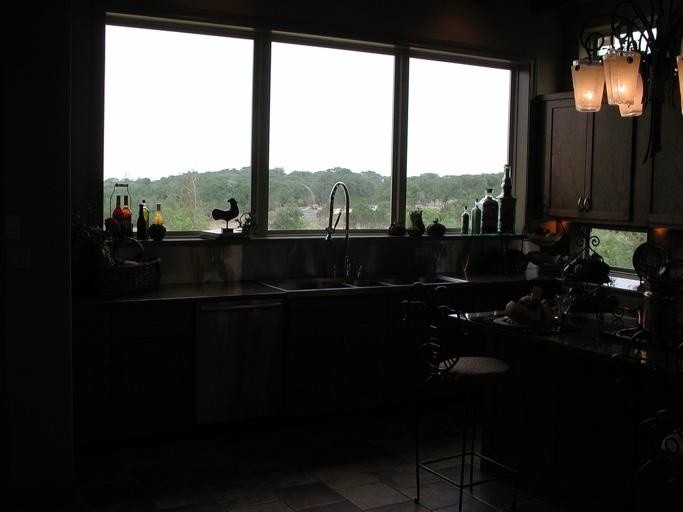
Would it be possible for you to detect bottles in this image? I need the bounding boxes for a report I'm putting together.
[112,194,132,227]
[137,197,164,240]
[460,164,516,236]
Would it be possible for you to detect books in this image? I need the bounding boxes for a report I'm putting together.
[199,229,246,243]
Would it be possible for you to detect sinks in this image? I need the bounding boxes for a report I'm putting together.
[339,277,394,289]
[257,278,356,293]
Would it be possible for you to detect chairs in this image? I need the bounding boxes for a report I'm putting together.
[398,280,533,510]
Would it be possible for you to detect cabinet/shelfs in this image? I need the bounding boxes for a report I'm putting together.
[473,290,511,430]
[285,292,386,423]
[635,80,682,230]
[73,296,197,450]
[616,323,683,512]
[386,290,472,428]
[196,298,286,428]
[606,294,645,318]
[537,92,642,228]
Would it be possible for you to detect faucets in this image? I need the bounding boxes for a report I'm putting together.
[325,181,352,280]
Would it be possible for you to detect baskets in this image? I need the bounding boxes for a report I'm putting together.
[89,238,160,293]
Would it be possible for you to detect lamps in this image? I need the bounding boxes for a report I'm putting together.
[564,1,681,117]
